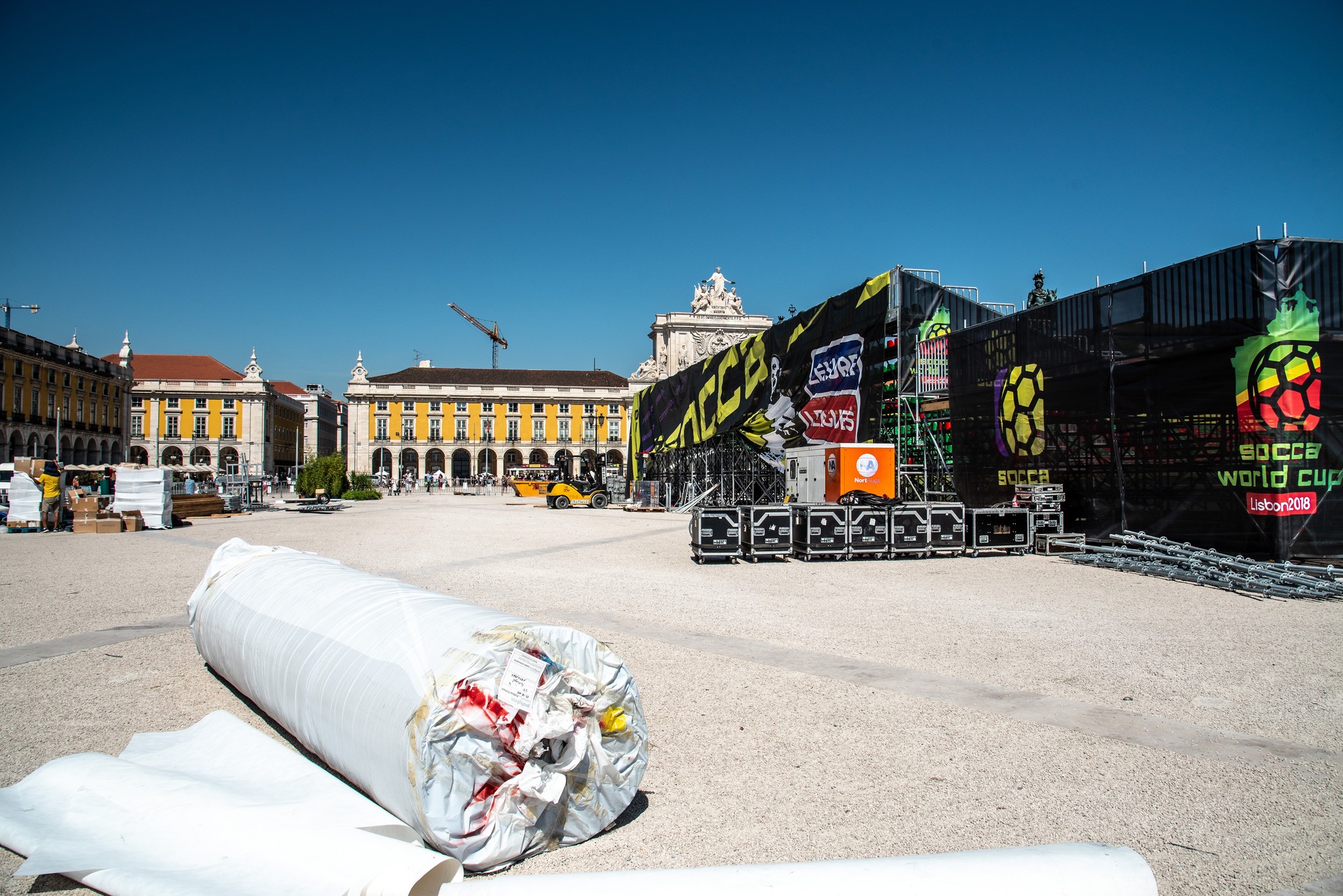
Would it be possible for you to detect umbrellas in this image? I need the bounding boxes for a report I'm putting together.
[64,463,227,482]
[479,472,493,475]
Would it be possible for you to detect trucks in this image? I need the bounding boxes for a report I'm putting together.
[784,442,895,503]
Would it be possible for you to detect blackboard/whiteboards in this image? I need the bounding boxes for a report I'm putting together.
[416,480,420,487]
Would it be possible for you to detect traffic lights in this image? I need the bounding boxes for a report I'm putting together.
[414,468,417,473]
[473,466,476,471]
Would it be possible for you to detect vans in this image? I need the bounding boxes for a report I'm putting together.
[0,462,14,503]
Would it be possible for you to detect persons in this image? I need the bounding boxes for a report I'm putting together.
[29,461,78,533]
[185,473,279,497]
[382,469,626,496]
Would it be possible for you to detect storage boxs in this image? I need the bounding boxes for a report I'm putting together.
[691,483,1087,565]
[6,456,142,534]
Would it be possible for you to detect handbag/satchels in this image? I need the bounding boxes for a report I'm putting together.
[267,486,272,493]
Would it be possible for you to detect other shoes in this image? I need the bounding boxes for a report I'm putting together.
[262,493,264,496]
[471,485,498,487]
[275,490,278,493]
[387,493,412,496]
[426,491,431,492]
[267,495,270,496]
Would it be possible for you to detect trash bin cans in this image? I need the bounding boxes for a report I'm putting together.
[425,479,427,487]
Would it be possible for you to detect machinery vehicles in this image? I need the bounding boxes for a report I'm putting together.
[546,453,631,510]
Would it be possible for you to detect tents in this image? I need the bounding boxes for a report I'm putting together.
[433,470,445,476]
[375,470,390,479]
[585,471,595,481]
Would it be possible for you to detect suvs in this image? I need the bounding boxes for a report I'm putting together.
[358,475,388,487]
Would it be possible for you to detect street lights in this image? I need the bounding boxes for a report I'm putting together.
[561,437,572,458]
[588,408,605,454]
[395,432,408,487]
[192,430,197,475]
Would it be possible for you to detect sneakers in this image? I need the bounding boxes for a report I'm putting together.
[53,528,59,532]
[40,528,49,533]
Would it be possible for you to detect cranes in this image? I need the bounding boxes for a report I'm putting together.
[447,302,509,369]
